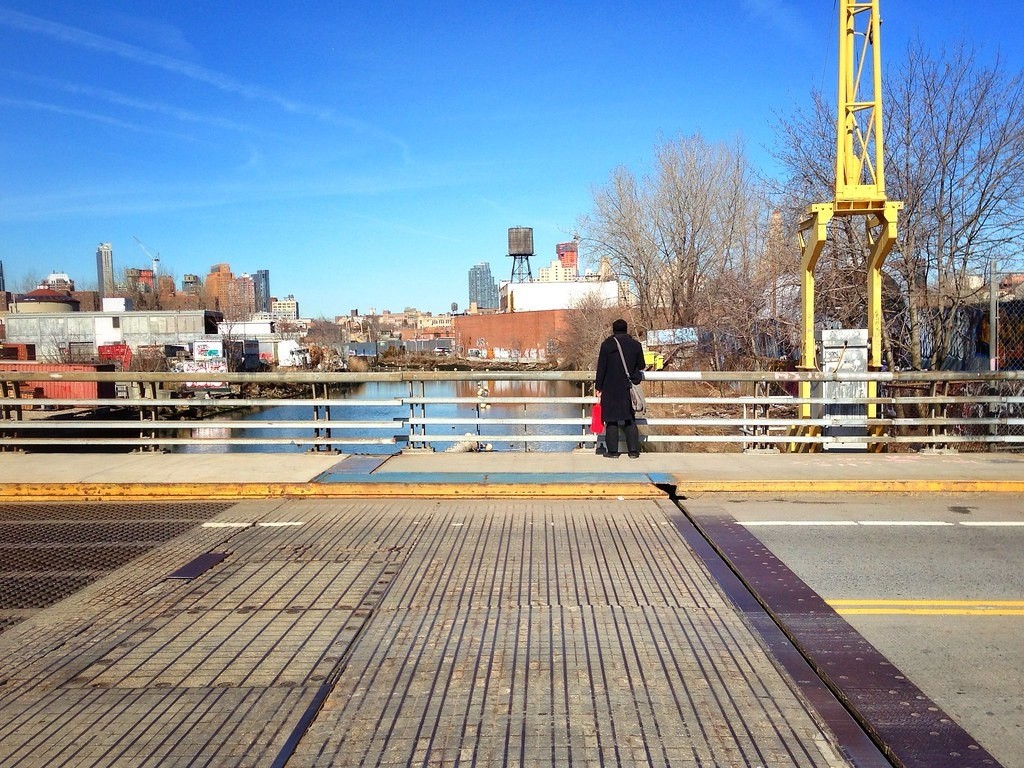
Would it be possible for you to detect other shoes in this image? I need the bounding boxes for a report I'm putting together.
[627,451,640,458]
[603,451,619,458]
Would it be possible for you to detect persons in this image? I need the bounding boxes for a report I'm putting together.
[595,319,646,458]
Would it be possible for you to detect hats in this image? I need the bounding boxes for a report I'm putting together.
[612,319,628,332]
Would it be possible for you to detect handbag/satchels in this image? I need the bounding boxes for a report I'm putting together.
[589,396,605,434]
[630,382,646,415]
